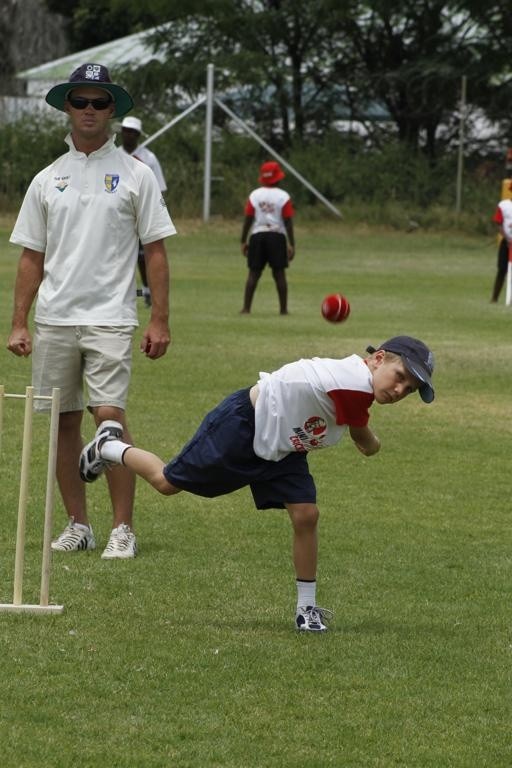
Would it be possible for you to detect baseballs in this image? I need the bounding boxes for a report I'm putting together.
[322,295,351,322]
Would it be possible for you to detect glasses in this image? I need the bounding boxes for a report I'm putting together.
[67,96,112,111]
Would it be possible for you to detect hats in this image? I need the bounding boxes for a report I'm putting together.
[46,63,135,119]
[112,116,150,139]
[377,335,434,404]
[258,161,286,185]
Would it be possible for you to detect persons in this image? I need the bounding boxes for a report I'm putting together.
[78,336,435,633]
[7,63,178,561]
[239,161,295,315]
[489,184,511,302]
[111,115,169,308]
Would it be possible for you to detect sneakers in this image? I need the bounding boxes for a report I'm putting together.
[100,523,141,559]
[78,420,124,482]
[294,604,334,631]
[51,517,97,553]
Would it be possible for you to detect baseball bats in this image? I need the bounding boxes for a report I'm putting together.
[506,243,511,305]
